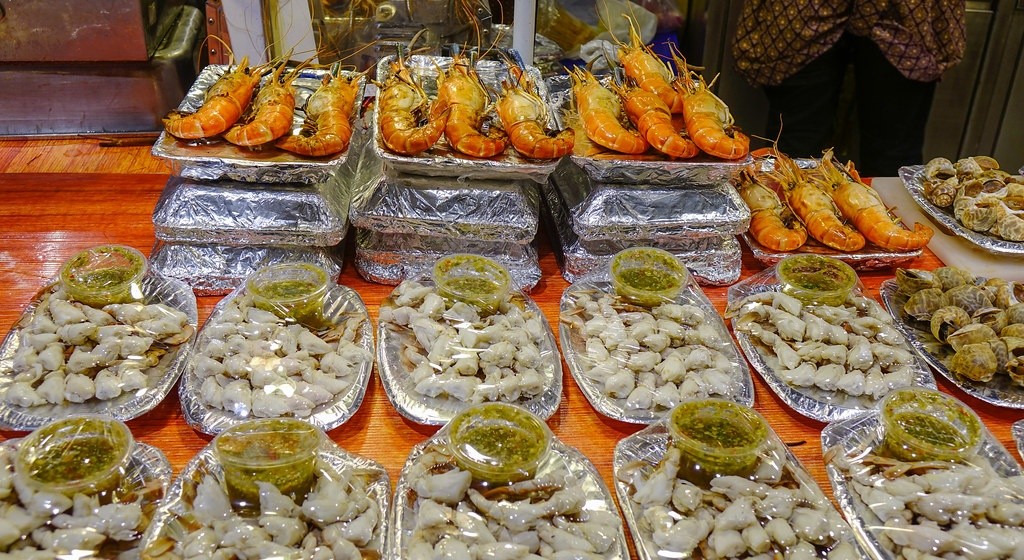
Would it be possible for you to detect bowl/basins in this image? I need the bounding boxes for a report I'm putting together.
[610,246,689,306]
[213,417,322,511]
[58,245,150,307]
[245,261,331,324]
[670,398,770,486]
[777,254,857,306]
[874,386,985,465]
[14,414,135,499]
[447,402,554,486]
[432,253,510,318]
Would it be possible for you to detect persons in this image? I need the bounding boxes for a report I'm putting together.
[731,0,967,178]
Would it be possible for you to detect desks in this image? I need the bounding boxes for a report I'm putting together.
[0,170,1024,560]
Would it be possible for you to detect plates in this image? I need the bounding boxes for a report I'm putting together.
[730,284,1024,559]
[178,285,374,430]
[137,437,390,559]
[380,288,561,425]
[152,63,1023,296]
[390,427,631,559]
[883,276,1024,462]
[561,278,867,559]
[0,434,172,560]
[0,276,200,431]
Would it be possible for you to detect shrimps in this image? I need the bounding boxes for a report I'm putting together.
[731,112,935,252]
[160,0,751,159]
